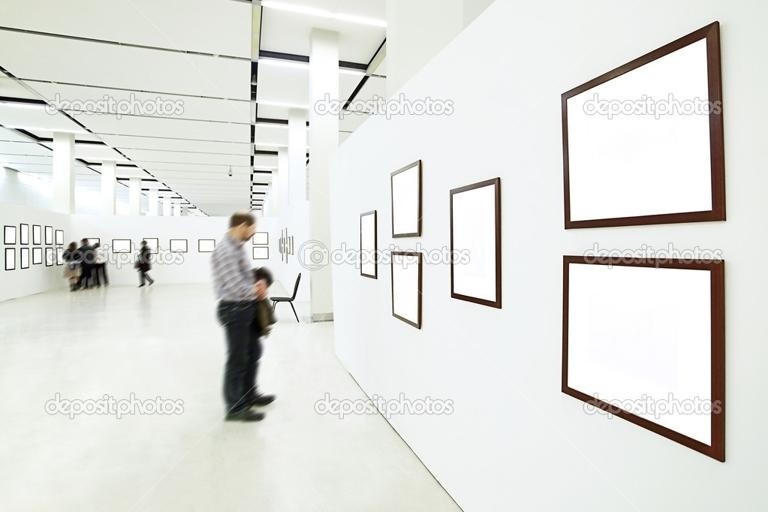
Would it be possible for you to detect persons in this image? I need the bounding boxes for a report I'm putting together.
[59,237,112,293]
[208,211,277,423]
[133,239,156,289]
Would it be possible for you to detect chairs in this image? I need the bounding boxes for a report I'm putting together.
[264,271,300,325]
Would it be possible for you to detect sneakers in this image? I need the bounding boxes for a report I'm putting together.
[226,409,264,421]
[245,395,274,407]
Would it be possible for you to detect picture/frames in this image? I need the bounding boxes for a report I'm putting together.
[449,177,501,308]
[86,238,217,255]
[391,251,419,329]
[561,21,728,230]
[391,160,422,237]
[4,222,64,270]
[250,228,294,265]
[561,256,725,461]
[359,210,378,279]
